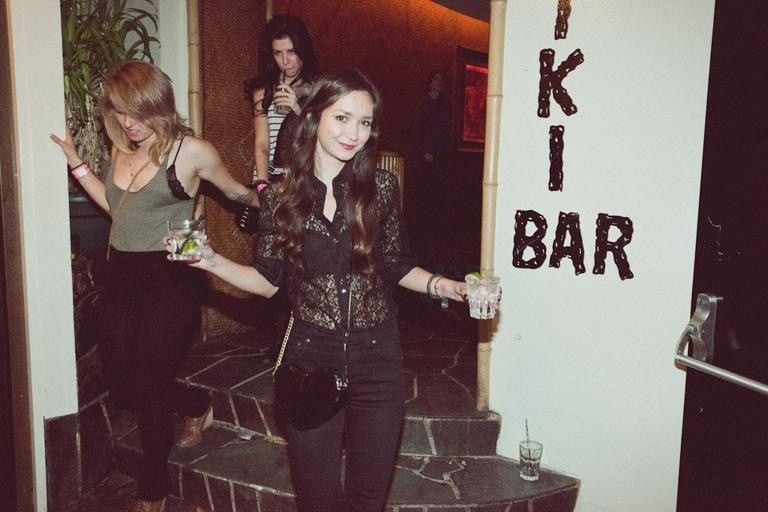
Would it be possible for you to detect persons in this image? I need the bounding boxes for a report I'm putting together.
[49,58,259,512]
[244,14,316,193]
[162,67,502,511]
[411,69,452,172]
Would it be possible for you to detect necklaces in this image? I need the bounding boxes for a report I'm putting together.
[128,154,149,178]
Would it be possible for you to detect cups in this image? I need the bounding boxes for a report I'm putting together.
[165,217,206,264]
[464,272,501,321]
[517,441,543,482]
[272,84,293,114]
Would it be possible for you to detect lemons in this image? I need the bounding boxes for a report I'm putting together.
[183,241,199,253]
[464,271,482,284]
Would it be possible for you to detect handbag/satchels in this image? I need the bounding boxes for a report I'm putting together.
[273,363,350,432]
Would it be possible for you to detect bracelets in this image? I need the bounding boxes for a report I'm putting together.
[255,179,268,193]
[434,276,449,310]
[71,161,90,179]
[426,273,441,297]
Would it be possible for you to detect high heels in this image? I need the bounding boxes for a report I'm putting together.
[173,398,215,448]
[130,497,167,512]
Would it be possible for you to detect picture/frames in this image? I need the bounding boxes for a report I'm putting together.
[454,44,489,155]
